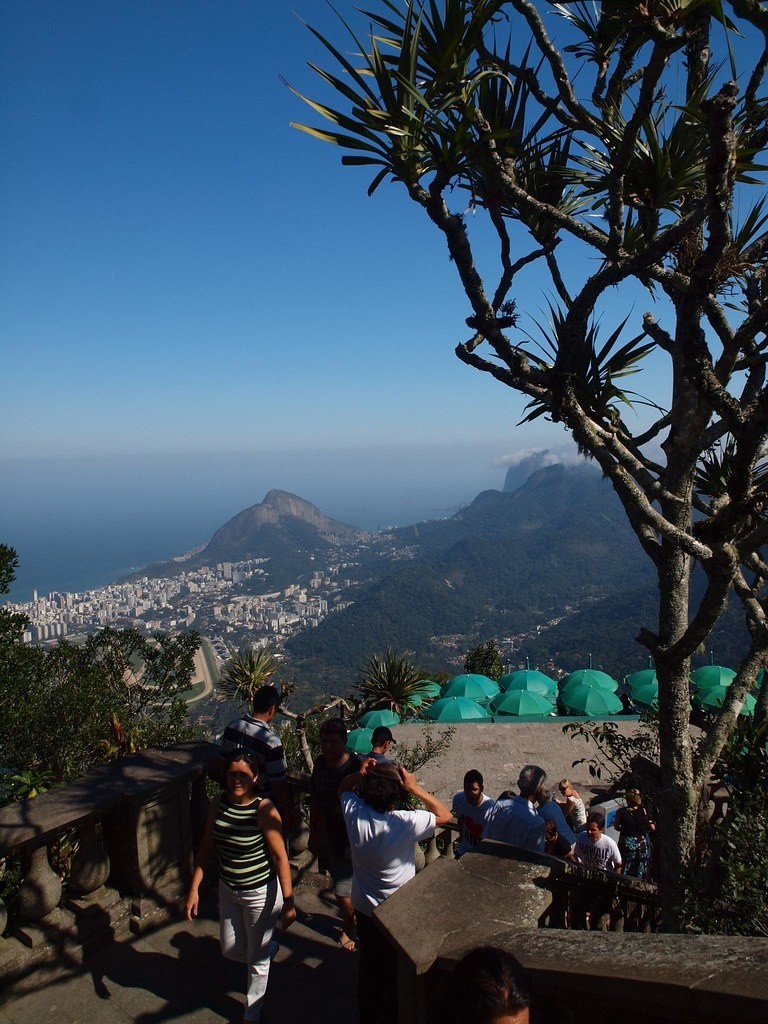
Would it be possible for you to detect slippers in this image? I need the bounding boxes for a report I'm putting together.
[338,922,356,952]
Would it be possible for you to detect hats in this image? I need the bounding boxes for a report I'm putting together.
[373,726,396,744]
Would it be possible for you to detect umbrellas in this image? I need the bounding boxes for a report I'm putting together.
[557,669,623,715]
[347,710,400,754]
[402,670,559,720]
[624,669,659,707]
[689,666,737,686]
[692,685,757,717]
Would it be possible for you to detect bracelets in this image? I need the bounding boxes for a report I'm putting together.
[284,896,294,901]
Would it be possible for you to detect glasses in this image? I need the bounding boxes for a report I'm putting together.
[229,749,255,762]
[560,786,568,790]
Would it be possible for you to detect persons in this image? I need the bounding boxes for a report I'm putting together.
[308,718,359,950]
[615,789,656,878]
[573,813,622,874]
[358,726,399,765]
[187,751,297,1024]
[433,947,534,1024]
[483,765,547,854]
[221,685,289,828]
[451,770,495,858]
[337,756,453,1024]
[497,790,516,800]
[536,780,587,860]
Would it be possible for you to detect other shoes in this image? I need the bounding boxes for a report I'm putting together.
[268,941,279,959]
